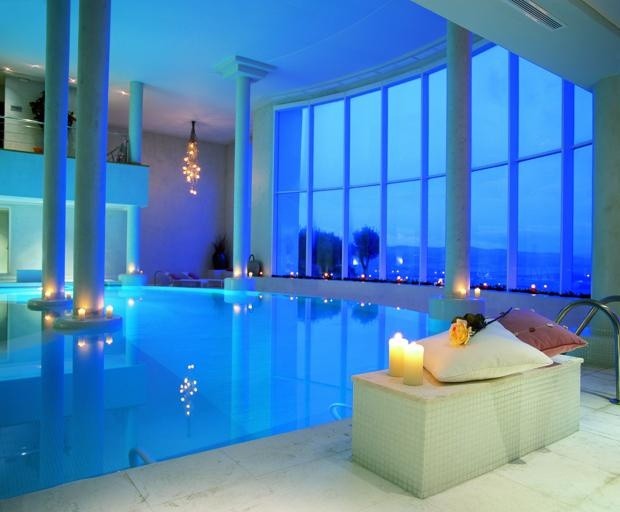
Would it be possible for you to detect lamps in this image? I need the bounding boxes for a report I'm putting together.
[177,363,199,433]
[182,121,202,195]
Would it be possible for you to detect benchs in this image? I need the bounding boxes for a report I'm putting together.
[351,356,585,498]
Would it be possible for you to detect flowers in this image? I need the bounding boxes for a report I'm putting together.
[448,308,513,348]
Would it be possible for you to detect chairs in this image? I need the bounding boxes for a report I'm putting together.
[154,270,223,288]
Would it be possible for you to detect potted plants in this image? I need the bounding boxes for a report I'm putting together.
[211,233,231,270]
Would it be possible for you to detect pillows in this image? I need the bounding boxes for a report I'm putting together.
[413,318,553,383]
[496,309,587,356]
[172,272,199,280]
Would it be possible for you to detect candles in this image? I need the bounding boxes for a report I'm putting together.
[474,288,480,297]
[67,291,71,298]
[389,338,408,377]
[106,333,113,344]
[403,342,424,387]
[106,305,112,317]
[78,335,86,348]
[44,312,50,319]
[78,308,86,320]
